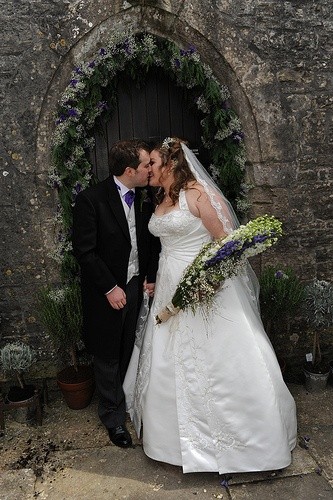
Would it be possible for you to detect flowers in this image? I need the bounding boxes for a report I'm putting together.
[303,279,333,330]
[156,213,283,324]
[0,342,44,377]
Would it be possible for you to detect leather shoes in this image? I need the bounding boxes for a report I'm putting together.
[109,424,132,448]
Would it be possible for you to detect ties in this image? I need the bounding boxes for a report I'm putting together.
[115,184,136,208]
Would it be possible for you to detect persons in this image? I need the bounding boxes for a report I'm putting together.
[122,136,297,474]
[72,138,162,448]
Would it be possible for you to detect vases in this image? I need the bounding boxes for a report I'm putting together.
[305,365,329,392]
[5,385,41,424]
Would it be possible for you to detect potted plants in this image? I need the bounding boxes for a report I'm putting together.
[258,265,305,383]
[37,252,95,411]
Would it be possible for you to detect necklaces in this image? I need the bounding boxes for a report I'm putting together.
[164,193,170,202]
